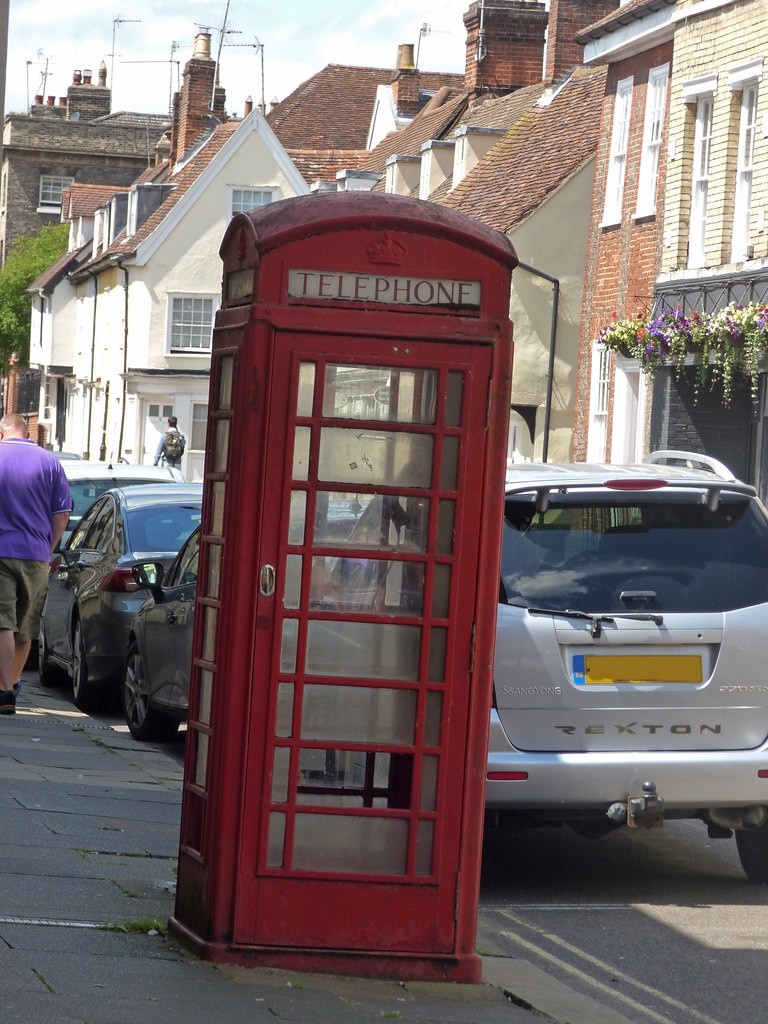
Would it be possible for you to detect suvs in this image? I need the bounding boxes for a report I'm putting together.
[309,448,767,883]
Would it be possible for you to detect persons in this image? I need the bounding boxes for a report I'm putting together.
[0,413,73,716]
[154,416,185,471]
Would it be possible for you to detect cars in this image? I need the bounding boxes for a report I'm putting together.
[120,505,367,743]
[36,482,202,713]
[41,462,186,552]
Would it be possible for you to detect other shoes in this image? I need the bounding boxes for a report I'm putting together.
[0,690,16,715]
[12,680,21,693]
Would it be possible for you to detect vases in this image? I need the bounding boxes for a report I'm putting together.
[708,336,724,349]
[666,344,686,356]
[726,332,745,349]
[618,341,636,358]
[687,339,704,354]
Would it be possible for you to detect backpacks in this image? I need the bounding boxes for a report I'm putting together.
[163,432,186,459]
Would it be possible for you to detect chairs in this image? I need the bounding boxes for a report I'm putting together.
[598,524,650,563]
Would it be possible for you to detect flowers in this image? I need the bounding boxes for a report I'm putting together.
[598,301,768,414]
